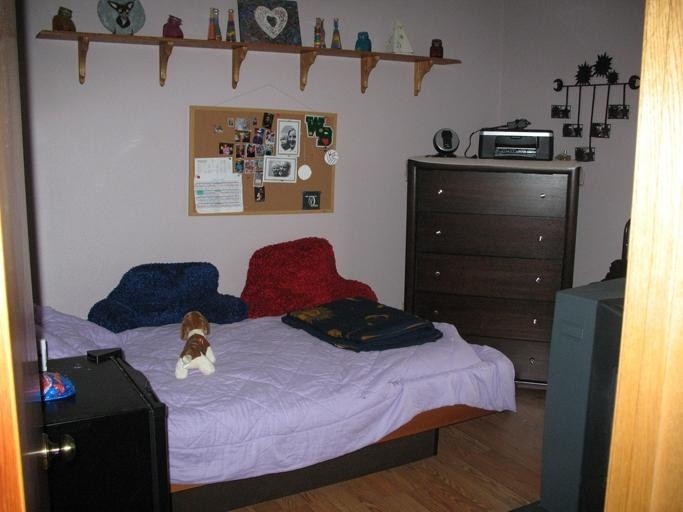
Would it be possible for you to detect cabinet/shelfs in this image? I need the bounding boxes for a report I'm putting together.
[405,155,581,390]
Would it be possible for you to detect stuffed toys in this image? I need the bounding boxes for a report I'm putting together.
[174,311,215,379]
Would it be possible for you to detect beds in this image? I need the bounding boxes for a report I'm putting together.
[35,298,518,512]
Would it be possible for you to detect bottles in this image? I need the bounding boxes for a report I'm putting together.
[331,17,342,49]
[51,4,76,30]
[355,32,371,52]
[225,8,236,43]
[320,20,327,49]
[314,17,322,49]
[162,14,184,40]
[212,10,222,41]
[207,8,214,40]
[429,39,446,58]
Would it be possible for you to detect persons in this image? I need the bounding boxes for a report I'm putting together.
[271,162,280,176]
[280,161,291,177]
[279,134,290,150]
[287,129,297,152]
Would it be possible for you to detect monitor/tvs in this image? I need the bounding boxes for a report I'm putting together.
[539,277,625,512]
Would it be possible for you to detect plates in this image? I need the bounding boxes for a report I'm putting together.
[95,1,145,37]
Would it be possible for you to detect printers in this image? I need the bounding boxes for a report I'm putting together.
[479,129,554,160]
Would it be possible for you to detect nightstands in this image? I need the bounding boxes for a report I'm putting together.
[40,349,171,511]
